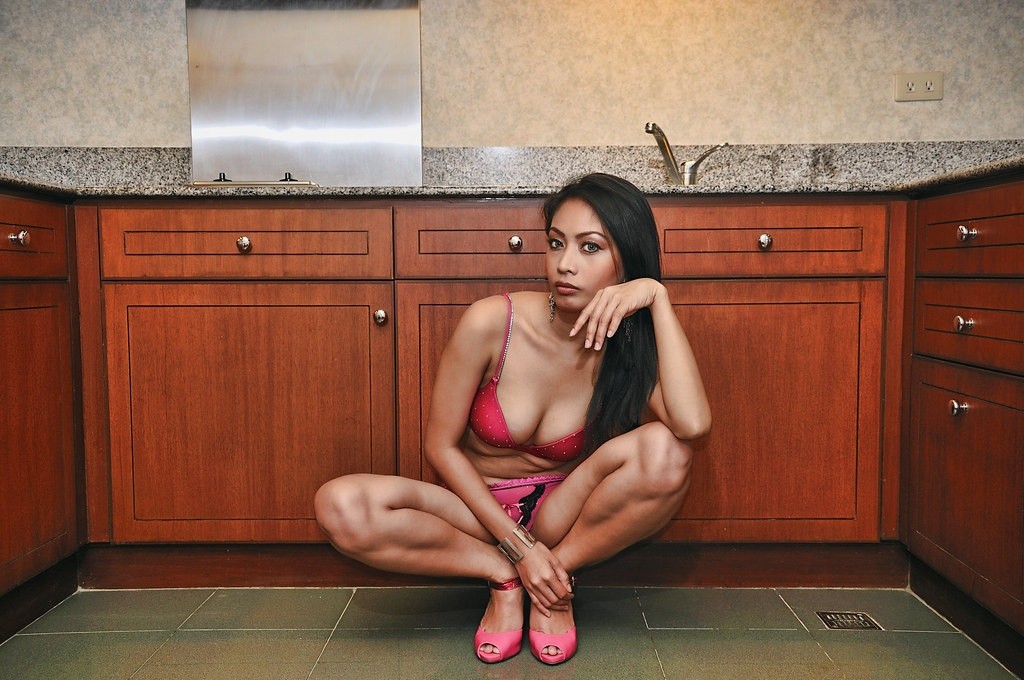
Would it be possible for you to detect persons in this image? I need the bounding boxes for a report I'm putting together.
[312,173,712,666]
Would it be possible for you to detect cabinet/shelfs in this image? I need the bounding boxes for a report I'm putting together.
[0,165,1024,680]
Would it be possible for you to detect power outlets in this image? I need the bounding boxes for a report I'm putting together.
[894,72,945,99]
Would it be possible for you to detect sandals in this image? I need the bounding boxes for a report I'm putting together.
[529,576,577,664]
[474,576,524,663]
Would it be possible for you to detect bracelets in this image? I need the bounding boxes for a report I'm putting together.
[497,524,537,565]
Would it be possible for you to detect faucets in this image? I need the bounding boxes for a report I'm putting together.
[646,121,729,185]
